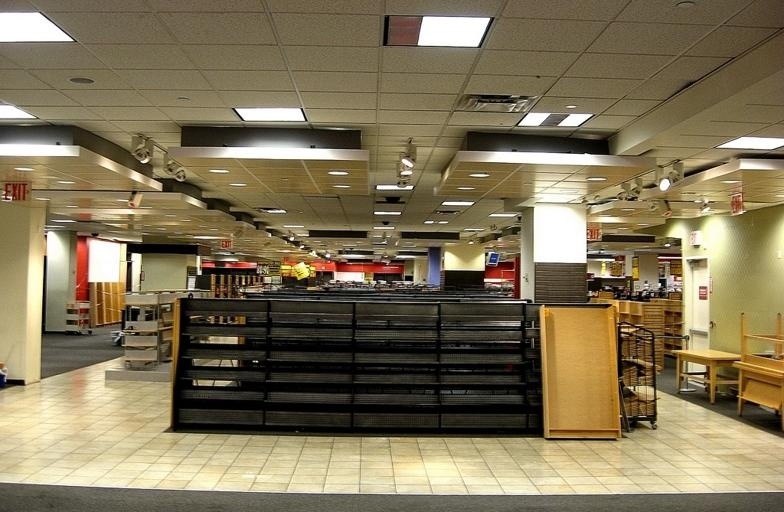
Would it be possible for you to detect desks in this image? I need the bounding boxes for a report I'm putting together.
[730,311,784,431]
[671,349,741,405]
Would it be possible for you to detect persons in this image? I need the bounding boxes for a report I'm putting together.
[374,279,397,288]
[598,280,681,302]
[334,280,340,288]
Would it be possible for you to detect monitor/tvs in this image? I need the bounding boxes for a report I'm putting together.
[486,251,500,265]
[263,275,317,288]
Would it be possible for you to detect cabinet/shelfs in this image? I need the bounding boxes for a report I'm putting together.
[89,282,542,437]
[591,297,683,386]
[65,303,92,334]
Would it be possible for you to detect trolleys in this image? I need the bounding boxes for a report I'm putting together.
[64,300,94,335]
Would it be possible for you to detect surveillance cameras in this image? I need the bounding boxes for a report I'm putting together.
[518,217,521,220]
[383,222,389,225]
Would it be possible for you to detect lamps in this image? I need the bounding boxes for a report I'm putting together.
[397,143,417,177]
